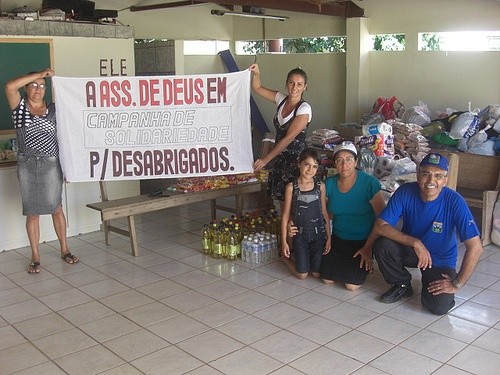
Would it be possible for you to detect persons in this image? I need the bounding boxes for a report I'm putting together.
[288,142,386,291]
[279,147,331,278]
[250,63,313,252]
[373,152,482,314]
[4,68,79,274]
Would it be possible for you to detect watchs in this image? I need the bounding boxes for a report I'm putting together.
[452,280,461,288]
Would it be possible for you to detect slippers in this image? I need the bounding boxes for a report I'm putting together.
[28,262,40,273]
[62,252,79,264]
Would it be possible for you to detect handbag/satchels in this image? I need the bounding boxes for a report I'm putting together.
[448,102,481,140]
[468,124,492,147]
[374,96,397,120]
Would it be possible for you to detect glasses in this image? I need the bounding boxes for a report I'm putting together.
[419,171,447,181]
[334,157,356,166]
[27,82,45,88]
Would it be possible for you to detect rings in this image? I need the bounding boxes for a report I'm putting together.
[369,260,371,261]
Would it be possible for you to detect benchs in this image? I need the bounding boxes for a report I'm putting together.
[86,181,262,256]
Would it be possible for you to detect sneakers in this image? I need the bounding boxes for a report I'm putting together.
[380,282,413,303]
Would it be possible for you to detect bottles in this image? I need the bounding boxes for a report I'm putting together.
[201,210,281,265]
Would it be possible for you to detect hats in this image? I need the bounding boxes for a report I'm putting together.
[420,152,449,172]
[333,141,357,156]
